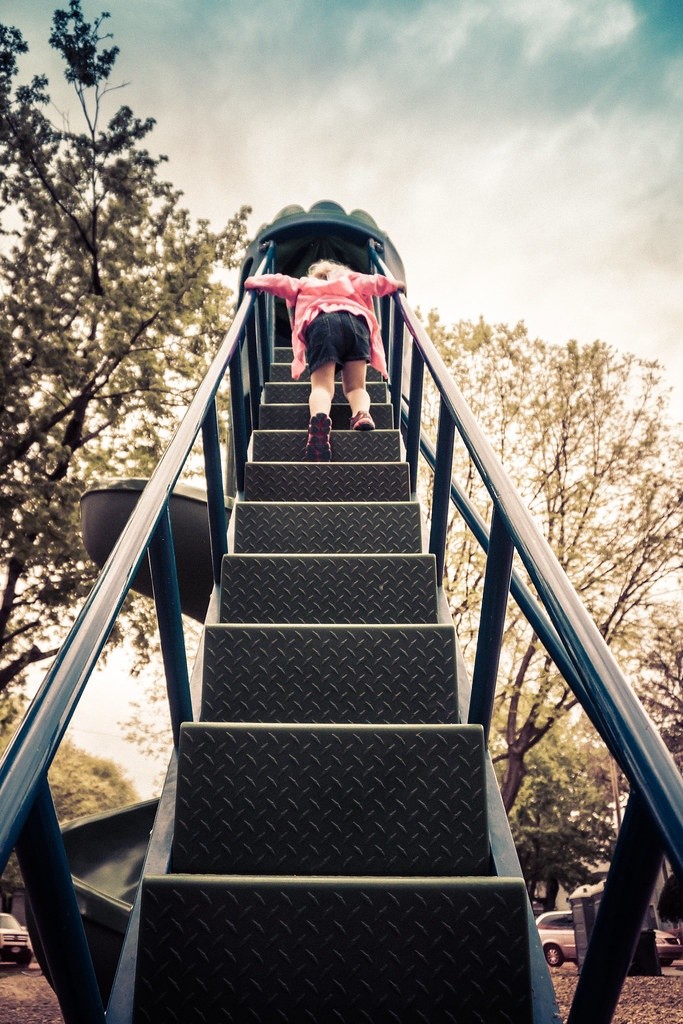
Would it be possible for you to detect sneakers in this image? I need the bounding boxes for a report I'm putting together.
[349,411,376,430]
[305,412,333,462]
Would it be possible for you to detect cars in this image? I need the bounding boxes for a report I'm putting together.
[535,910,683,967]
[0,913,33,967]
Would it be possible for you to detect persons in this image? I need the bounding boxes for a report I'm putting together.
[243,259,409,464]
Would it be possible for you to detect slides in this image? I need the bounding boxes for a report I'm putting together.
[19,480,238,979]
